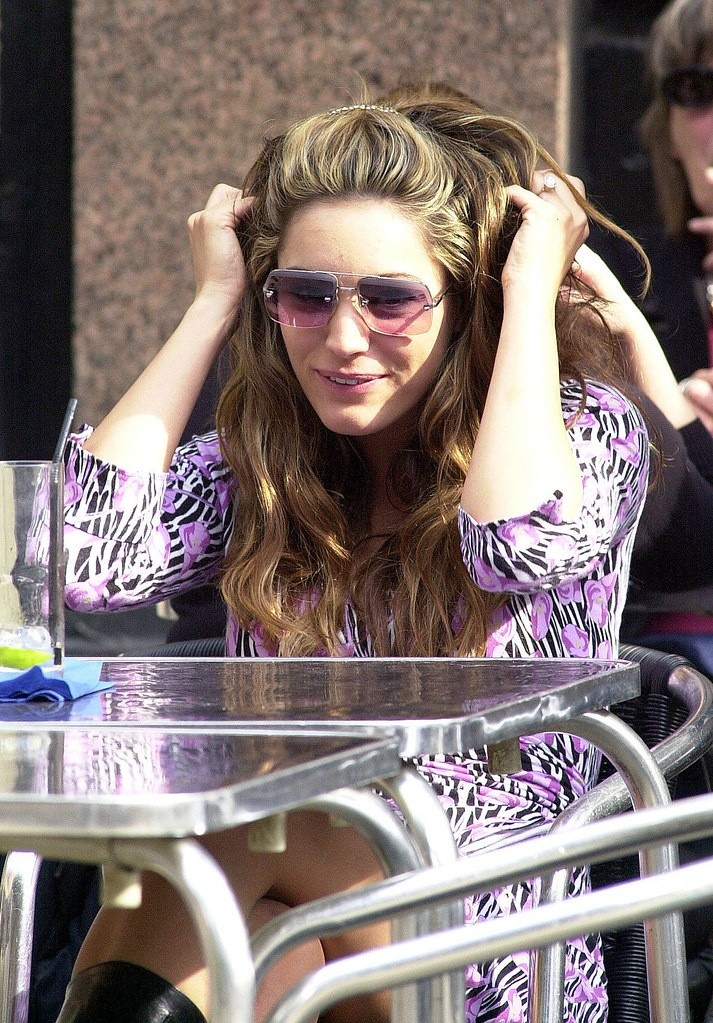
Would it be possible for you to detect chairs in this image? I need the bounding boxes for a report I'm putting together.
[28,637,712,1022]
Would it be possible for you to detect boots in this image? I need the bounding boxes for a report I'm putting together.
[56,959,208,1022]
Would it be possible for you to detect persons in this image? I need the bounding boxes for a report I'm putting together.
[24,95,653,1023]
[552,0,713,1002]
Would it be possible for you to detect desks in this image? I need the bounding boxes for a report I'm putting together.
[0,654,690,1023]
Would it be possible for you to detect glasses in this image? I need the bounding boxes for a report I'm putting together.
[263,267,455,338]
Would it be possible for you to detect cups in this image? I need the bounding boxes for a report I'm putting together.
[0,461,65,673]
